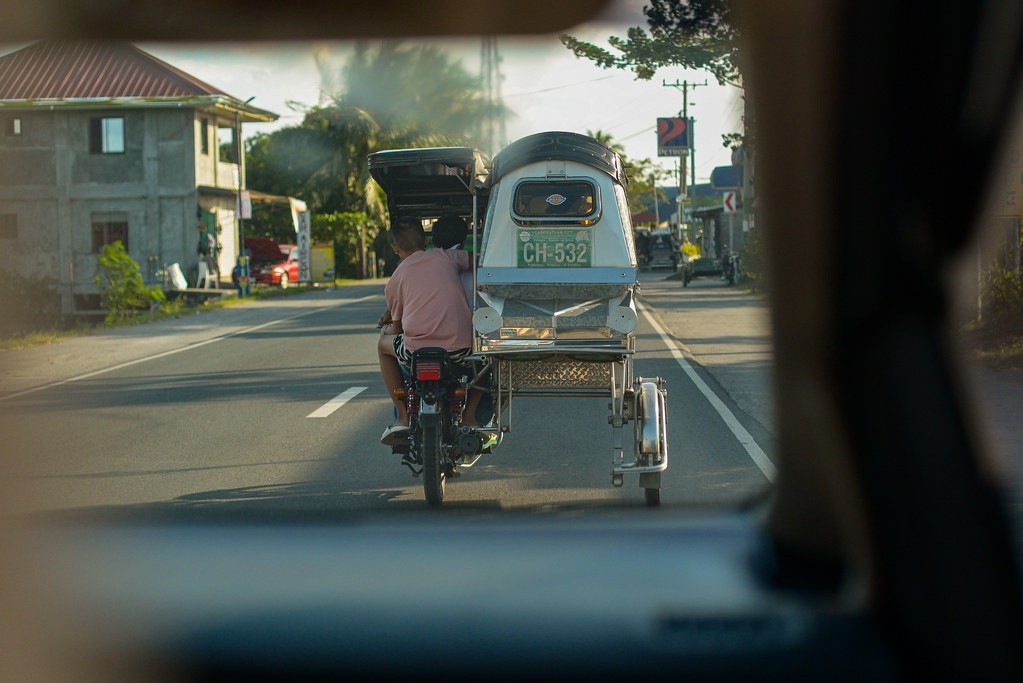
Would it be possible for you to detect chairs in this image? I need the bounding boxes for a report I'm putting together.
[195,261,219,289]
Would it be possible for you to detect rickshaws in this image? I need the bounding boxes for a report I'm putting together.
[366,130,669,506]
[633,226,739,289]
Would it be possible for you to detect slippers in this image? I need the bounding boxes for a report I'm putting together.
[379,424,410,445]
[484,433,498,448]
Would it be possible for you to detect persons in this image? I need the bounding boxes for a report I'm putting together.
[635,233,653,265]
[196,223,215,290]
[378,214,498,467]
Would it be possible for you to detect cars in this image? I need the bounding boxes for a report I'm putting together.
[244,238,299,290]
